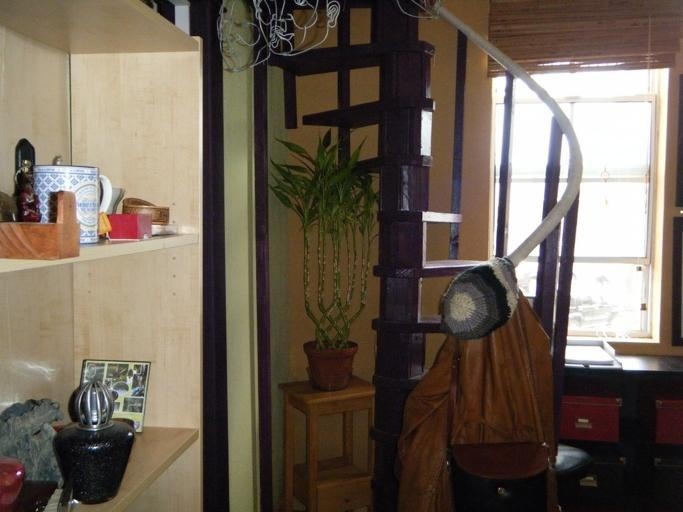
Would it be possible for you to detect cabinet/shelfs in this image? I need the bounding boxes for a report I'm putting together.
[276,375,377,511]
[0,2,206,511]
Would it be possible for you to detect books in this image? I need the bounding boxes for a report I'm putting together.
[551,343,613,366]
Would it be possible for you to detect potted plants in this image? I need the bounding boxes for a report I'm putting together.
[266,123,391,391]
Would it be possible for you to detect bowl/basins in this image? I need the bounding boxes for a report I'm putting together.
[0,457,25,512]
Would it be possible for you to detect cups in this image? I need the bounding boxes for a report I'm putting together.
[31,162,113,246]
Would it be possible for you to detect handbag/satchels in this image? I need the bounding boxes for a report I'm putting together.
[445,439,553,512]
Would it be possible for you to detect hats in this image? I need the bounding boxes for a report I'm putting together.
[438,255,520,341]
